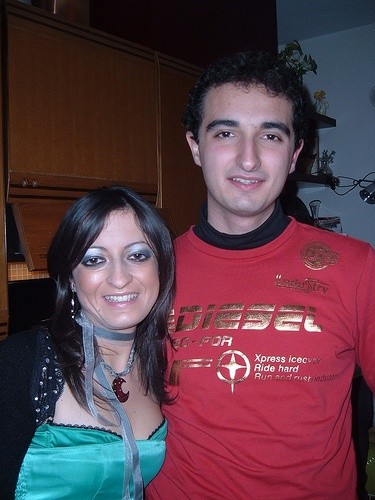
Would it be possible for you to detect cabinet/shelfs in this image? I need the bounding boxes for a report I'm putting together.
[0,0,341,343]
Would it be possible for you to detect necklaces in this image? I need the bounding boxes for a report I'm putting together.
[97,338,137,403]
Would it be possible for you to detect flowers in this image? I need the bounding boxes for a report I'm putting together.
[312,91,326,99]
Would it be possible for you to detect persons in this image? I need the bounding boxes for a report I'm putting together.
[0,185,176,499]
[143,50,374,500]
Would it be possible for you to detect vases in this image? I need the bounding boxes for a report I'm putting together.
[316,158,334,176]
[313,99,329,115]
